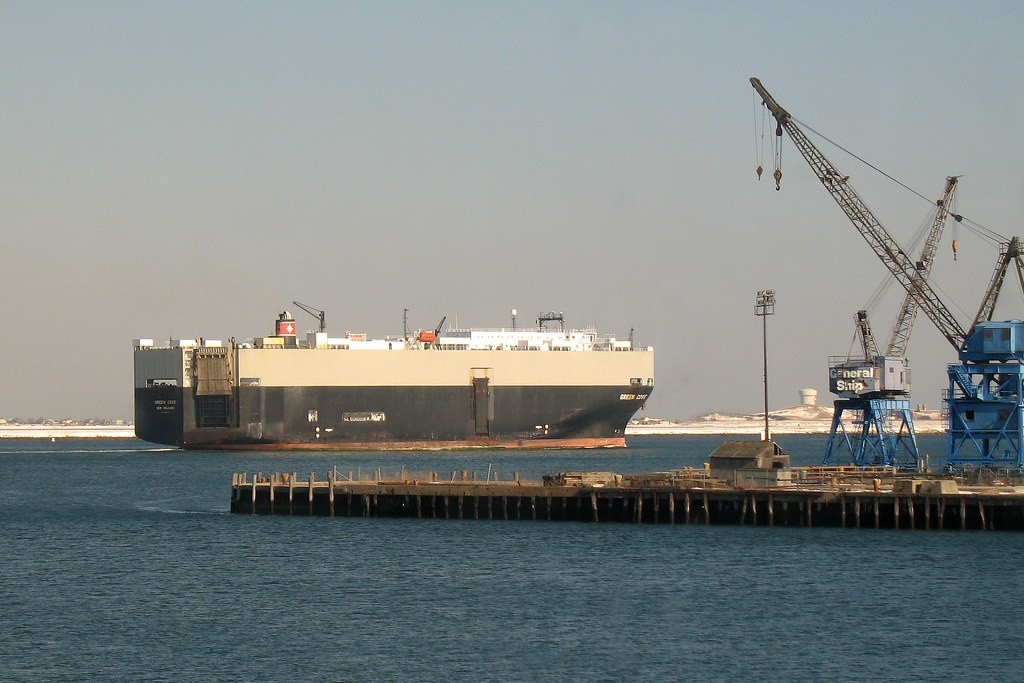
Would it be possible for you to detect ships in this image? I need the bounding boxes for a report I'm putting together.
[130,299,655,452]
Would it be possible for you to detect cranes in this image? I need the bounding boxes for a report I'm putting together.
[746,76,1024,477]
